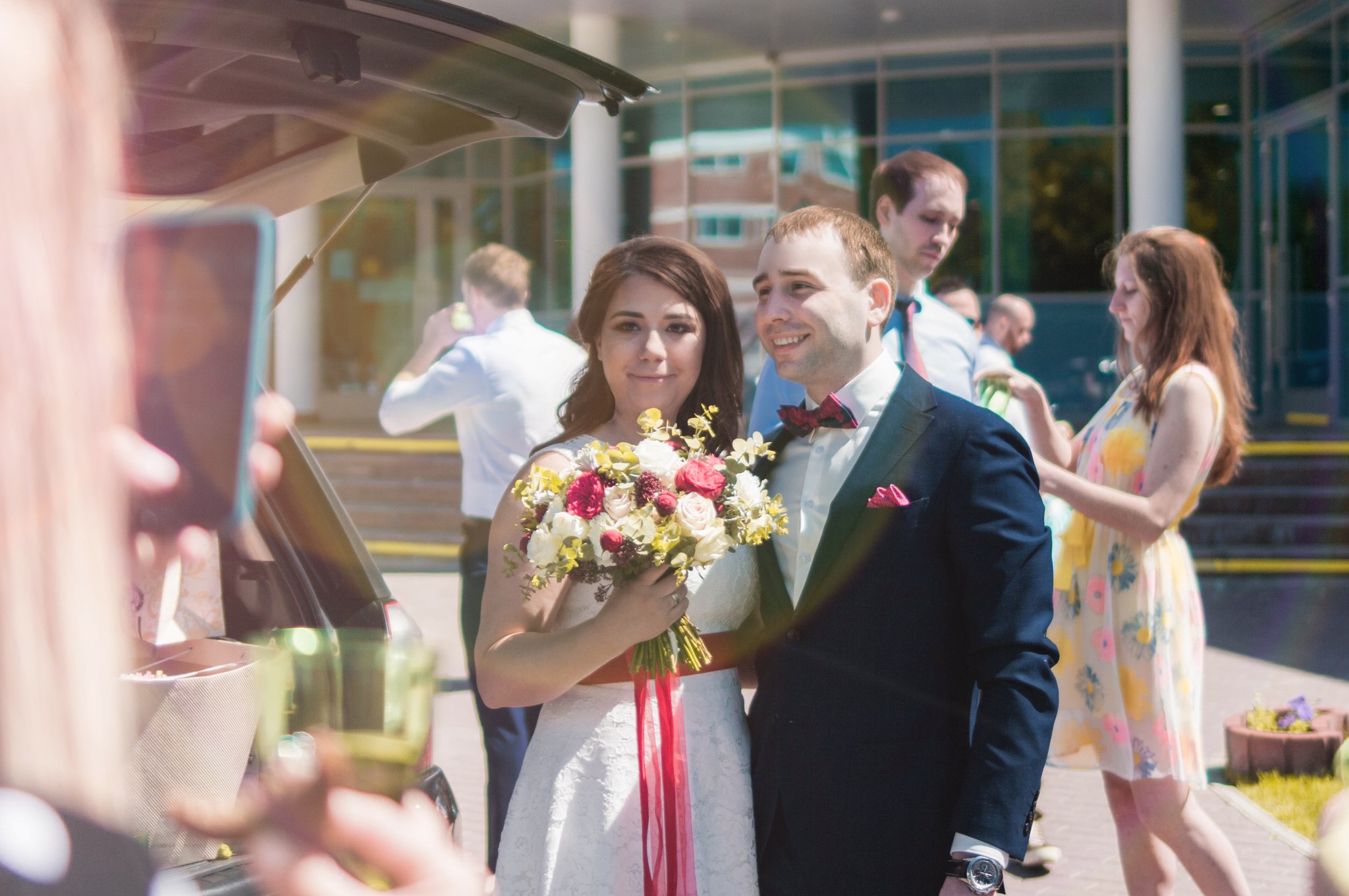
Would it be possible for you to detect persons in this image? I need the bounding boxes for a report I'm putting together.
[377,244,589,878]
[741,205,1060,896]
[0,0,459,896]
[473,232,761,896]
[736,147,1072,571]
[974,222,1258,896]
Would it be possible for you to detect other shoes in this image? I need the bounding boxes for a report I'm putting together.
[1008,810,1061,866]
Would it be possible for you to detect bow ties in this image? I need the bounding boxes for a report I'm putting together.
[776,392,858,438]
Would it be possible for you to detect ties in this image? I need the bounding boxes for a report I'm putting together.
[895,299,930,383]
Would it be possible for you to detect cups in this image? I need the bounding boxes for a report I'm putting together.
[252,627,436,891]
[452,302,474,332]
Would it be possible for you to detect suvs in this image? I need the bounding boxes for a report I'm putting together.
[107,0,658,895]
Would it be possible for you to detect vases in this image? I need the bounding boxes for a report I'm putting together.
[1224,708,1349,784]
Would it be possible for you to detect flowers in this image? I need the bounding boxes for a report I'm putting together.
[493,403,790,675]
[1245,691,1316,733]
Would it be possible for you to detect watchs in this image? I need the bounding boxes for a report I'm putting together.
[944,855,1003,895]
